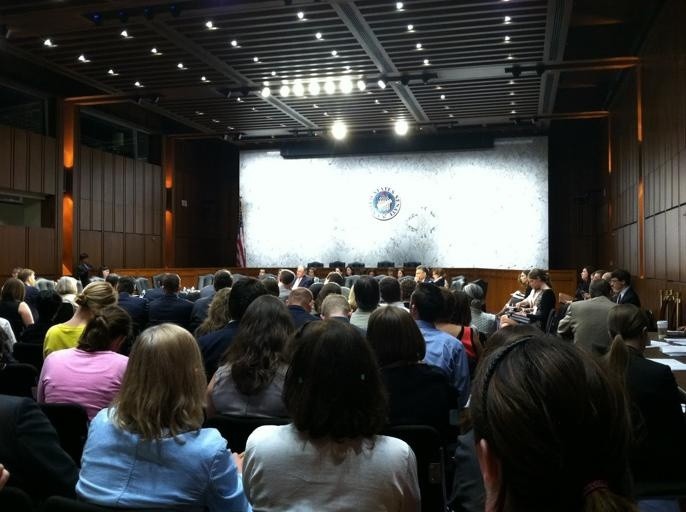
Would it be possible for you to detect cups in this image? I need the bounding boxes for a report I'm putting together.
[657,320,668,342]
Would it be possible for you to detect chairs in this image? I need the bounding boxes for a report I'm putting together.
[37,401,89,468]
[546,307,557,335]
[376,260,395,268]
[379,423,449,512]
[12,342,44,373]
[403,261,422,268]
[329,261,346,268]
[203,414,292,454]
[307,260,324,267]
[8,362,40,400]
[347,262,365,269]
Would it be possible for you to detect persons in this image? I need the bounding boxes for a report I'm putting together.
[148,273,195,323]
[345,266,354,276]
[21,290,62,343]
[415,265,434,285]
[611,269,641,306]
[335,266,343,275]
[497,270,530,314]
[557,279,626,346]
[17,268,40,303]
[258,269,268,277]
[499,273,527,314]
[283,287,322,326]
[75,324,251,512]
[242,320,422,512]
[591,273,595,281]
[315,282,342,316]
[0,327,74,512]
[463,284,497,333]
[409,283,471,405]
[42,281,124,358]
[429,268,445,287]
[0,278,34,324]
[368,269,377,276]
[350,276,383,323]
[500,268,554,329]
[37,305,132,421]
[578,267,594,299]
[196,278,275,380]
[309,268,319,284]
[205,295,298,416]
[144,274,171,299]
[472,333,686,512]
[118,277,149,322]
[594,271,605,281]
[100,265,112,279]
[0,317,17,352]
[192,287,234,338]
[293,265,313,290]
[77,253,96,279]
[365,306,461,426]
[399,278,416,302]
[398,269,405,279]
[603,304,686,498]
[300,294,372,361]
[323,273,342,287]
[194,271,235,323]
[106,275,119,291]
[601,270,612,282]
[380,277,410,312]
[278,269,294,300]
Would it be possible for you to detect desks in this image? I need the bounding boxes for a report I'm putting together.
[641,328,686,398]
